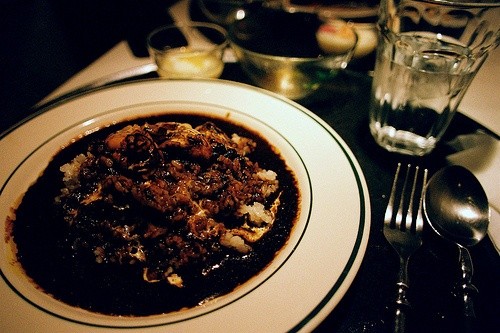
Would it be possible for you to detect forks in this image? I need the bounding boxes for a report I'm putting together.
[384,162,429,333]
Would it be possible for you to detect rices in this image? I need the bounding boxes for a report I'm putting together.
[57,121,278,289]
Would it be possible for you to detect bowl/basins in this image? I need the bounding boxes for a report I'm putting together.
[228,10,358,100]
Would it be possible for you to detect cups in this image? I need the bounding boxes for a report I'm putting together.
[146,22,229,79]
[369,0,500,157]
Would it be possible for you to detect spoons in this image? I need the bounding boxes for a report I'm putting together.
[423,164,490,333]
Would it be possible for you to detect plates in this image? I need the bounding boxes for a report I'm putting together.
[1,77,371,331]
